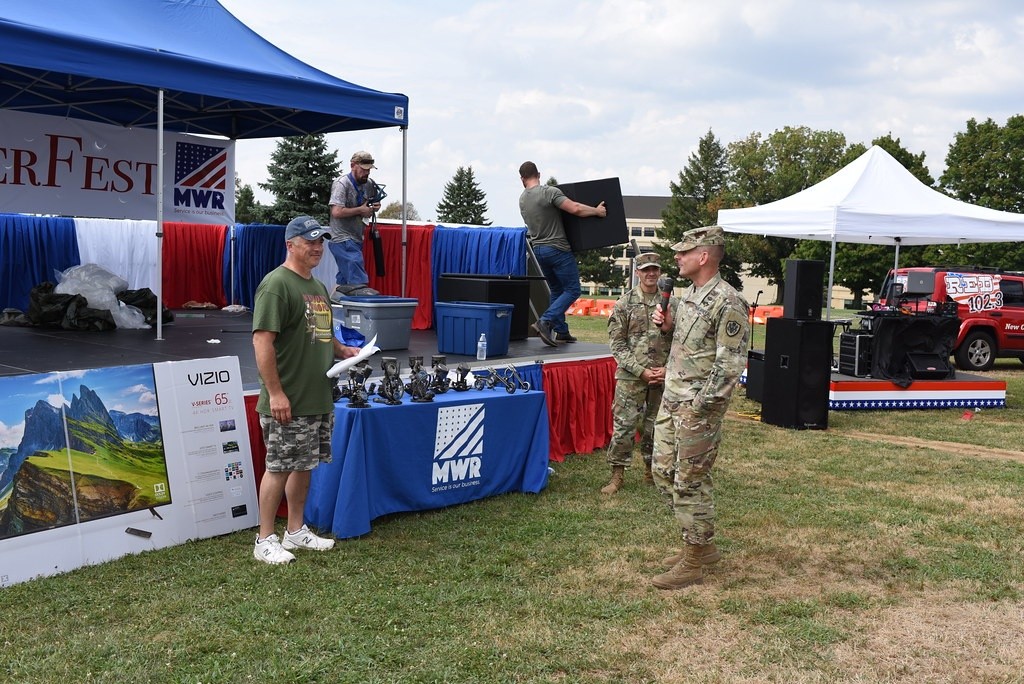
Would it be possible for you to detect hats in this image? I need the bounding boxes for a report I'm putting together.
[350,151,378,170]
[635,252,662,270]
[670,225,725,251]
[285,215,331,241]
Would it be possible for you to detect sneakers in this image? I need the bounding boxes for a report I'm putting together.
[253,532,296,565]
[556,331,578,343]
[282,524,335,552]
[531,319,559,347]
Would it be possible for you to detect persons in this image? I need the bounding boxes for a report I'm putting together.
[252,215,361,564]
[328,151,382,286]
[600,251,680,493]
[652,225,751,589]
[519,161,607,346]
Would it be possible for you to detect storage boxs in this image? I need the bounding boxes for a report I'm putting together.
[327,294,418,351]
[435,299,515,358]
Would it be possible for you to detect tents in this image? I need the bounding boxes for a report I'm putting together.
[1,0,410,341]
[718,145,1024,323]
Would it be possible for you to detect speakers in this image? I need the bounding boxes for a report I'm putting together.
[746,258,835,431]
[902,352,950,379]
[438,277,531,340]
[555,178,628,250]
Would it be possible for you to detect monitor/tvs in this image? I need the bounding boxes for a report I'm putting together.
[0,363,173,541]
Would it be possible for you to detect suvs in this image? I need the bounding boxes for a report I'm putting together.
[877,265,1024,371]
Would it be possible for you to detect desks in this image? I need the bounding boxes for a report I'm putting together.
[304,384,550,538]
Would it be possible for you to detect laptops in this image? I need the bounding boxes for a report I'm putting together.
[896,271,935,298]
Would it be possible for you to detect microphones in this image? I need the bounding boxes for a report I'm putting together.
[655,279,674,327]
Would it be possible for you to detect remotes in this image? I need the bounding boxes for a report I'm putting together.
[124,526,152,538]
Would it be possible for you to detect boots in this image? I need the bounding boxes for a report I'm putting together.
[601,465,624,493]
[652,545,704,588]
[645,464,655,485]
[662,540,721,567]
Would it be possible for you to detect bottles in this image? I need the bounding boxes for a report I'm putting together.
[547,467,555,474]
[476,333,487,360]
